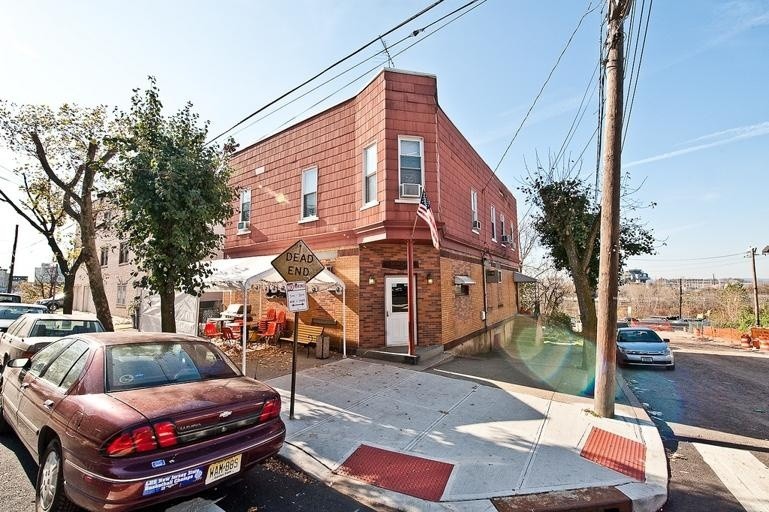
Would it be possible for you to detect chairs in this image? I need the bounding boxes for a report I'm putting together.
[199,308,327,360]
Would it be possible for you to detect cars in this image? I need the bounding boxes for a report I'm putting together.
[624,316,704,330]
[0,329,286,510]
[0,293,21,305]
[616,319,628,330]
[1,311,108,371]
[36,292,64,312]
[616,326,675,371]
[0,302,47,335]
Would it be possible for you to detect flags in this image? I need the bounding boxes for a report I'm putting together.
[417,189,440,250]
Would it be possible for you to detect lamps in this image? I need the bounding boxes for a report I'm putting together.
[366,273,377,286]
[425,272,434,285]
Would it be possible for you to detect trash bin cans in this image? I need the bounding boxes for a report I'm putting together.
[316,336,330,359]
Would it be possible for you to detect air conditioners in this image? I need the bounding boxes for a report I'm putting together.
[400,182,421,199]
[236,220,250,233]
[472,219,482,232]
[501,234,512,246]
[485,267,504,285]
